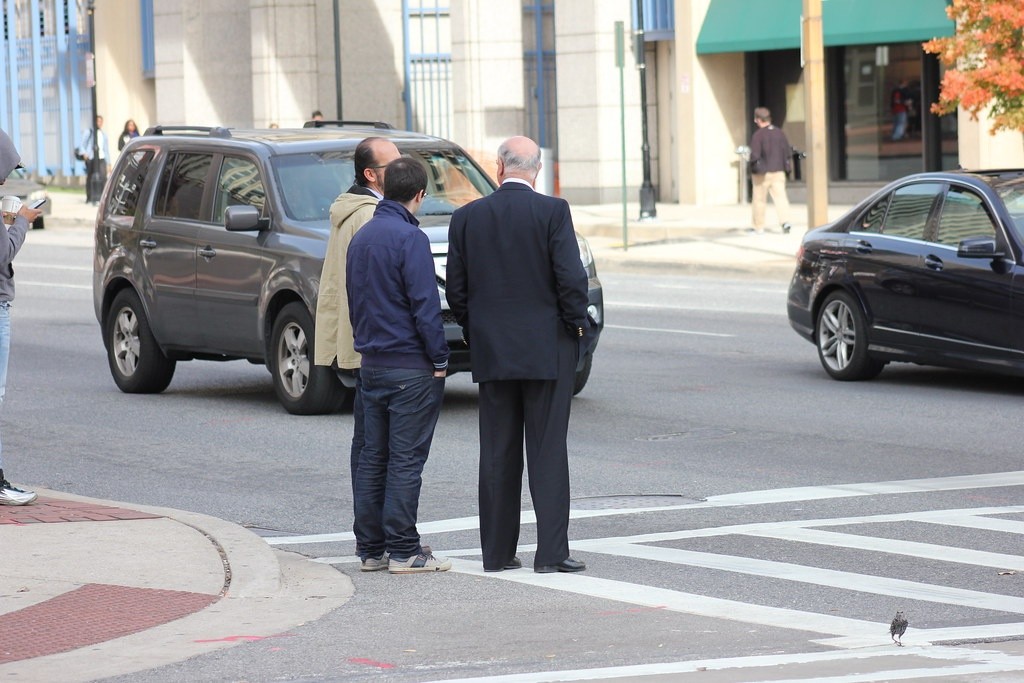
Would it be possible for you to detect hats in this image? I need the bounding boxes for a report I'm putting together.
[15,160,24,171]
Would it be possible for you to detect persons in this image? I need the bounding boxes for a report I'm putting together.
[740,107,793,234]
[78,116,112,205]
[312,110,324,129]
[268,122,280,129]
[444,136,593,575]
[313,136,433,558]
[0,126,42,507]
[118,119,141,152]
[344,157,452,574]
[888,75,914,143]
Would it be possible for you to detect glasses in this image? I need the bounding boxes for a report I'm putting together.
[365,165,386,171]
[423,191,428,198]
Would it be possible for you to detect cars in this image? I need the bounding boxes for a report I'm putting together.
[1,159,52,229]
[788,165,1024,396]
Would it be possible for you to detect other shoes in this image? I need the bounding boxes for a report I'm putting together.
[780,222,791,234]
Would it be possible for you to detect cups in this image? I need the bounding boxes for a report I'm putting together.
[1,194,22,225]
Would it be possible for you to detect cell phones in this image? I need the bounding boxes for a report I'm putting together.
[28,200,46,209]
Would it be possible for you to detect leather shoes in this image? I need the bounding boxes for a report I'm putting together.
[485,555,520,573]
[534,556,586,575]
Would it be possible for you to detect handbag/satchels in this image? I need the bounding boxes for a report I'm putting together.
[74,147,85,160]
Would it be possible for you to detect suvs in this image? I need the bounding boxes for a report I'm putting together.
[92,115,604,418]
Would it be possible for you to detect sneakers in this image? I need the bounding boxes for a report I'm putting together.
[359,550,389,572]
[387,552,450,574]
[0,468,38,507]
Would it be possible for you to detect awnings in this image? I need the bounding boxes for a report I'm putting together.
[695,0,956,56]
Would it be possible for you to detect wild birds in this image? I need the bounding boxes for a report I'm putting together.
[888,610,908,648]
